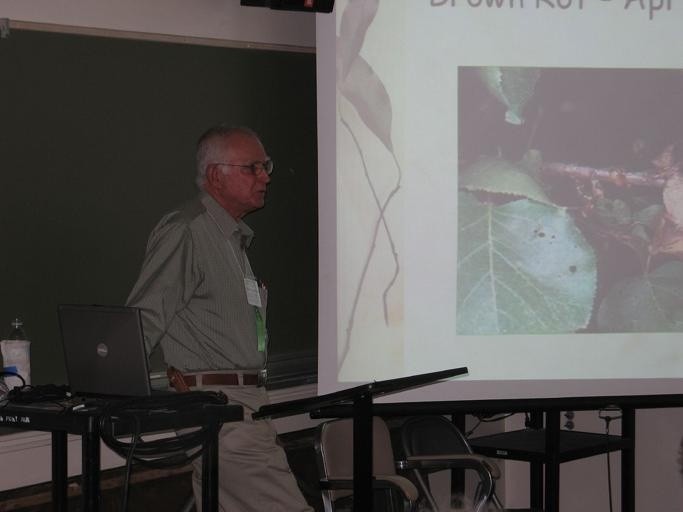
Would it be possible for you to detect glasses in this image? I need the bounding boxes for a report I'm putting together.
[217,160,273,175]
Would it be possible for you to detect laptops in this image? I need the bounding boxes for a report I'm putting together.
[58,305,219,401]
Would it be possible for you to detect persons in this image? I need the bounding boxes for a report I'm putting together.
[124,126,326,511]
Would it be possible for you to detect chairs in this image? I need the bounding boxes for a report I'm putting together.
[314,417,505,512]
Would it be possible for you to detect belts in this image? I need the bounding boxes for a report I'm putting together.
[169,369,268,387]
